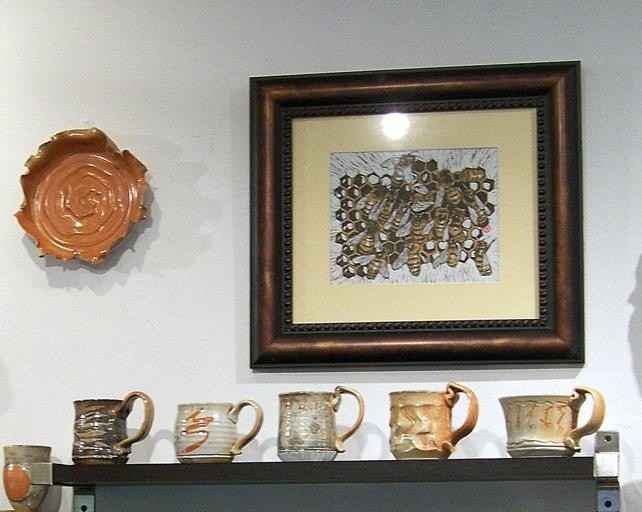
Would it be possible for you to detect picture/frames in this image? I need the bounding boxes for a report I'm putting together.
[248,59,586,369]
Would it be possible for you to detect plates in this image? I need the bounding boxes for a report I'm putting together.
[16,127,149,267]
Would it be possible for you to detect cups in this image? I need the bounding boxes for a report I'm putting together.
[275,385,366,463]
[387,382,481,460]
[499,388,613,457]
[72,392,156,464]
[174,399,265,464]
[4,444,51,512]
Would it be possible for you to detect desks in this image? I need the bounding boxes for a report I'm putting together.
[53,456,597,512]
